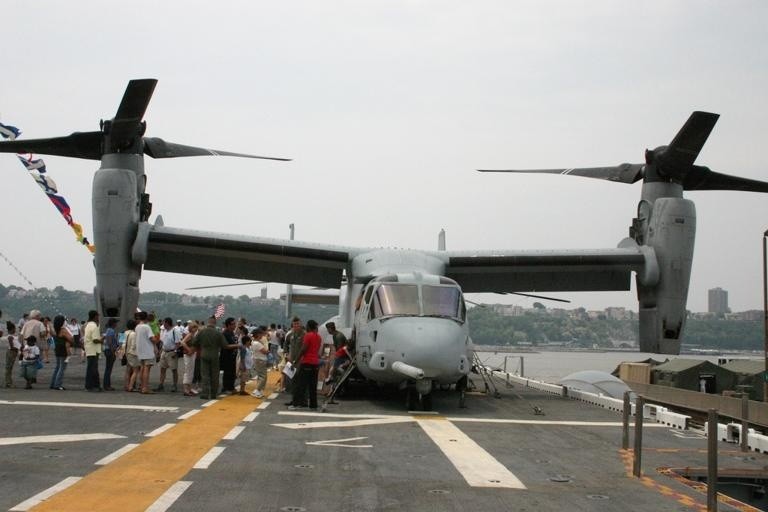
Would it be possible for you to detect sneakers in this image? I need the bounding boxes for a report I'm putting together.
[49,386,65,390]
[25,384,32,389]
[184,387,216,399]
[153,384,163,391]
[85,385,113,392]
[171,384,177,391]
[284,400,317,408]
[7,384,16,388]
[124,384,149,393]
[223,388,264,398]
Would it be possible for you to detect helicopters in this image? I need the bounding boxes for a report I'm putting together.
[1,79,768,410]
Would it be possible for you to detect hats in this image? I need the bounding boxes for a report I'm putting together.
[209,314,214,318]
[25,336,35,341]
[326,322,334,327]
[108,318,119,323]
[293,316,299,321]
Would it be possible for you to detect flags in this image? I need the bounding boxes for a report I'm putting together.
[0,123,95,254]
[206,302,225,321]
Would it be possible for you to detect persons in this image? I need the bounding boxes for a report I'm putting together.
[325,322,349,396]
[283,316,324,409]
[1,310,85,390]
[122,311,288,400]
[84,310,120,392]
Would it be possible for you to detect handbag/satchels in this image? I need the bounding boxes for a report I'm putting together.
[121,354,127,365]
[178,346,183,357]
